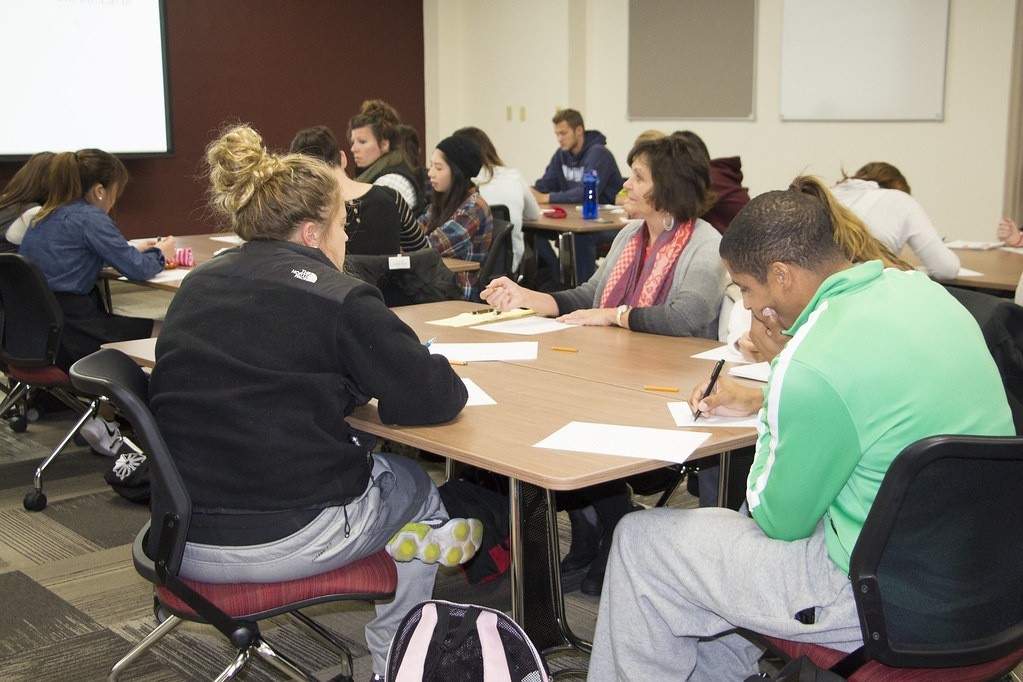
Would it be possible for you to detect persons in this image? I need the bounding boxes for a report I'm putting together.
[347,101,427,218]
[997,217,1023,247]
[529,109,624,288]
[399,135,495,302]
[18,149,176,457]
[453,125,544,284]
[671,131,751,236]
[150,116,483,682]
[829,162,961,280]
[0,151,61,255]
[615,128,667,206]
[479,135,733,341]
[728,164,915,363]
[289,125,430,256]
[585,189,1016,682]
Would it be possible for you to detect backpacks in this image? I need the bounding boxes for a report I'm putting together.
[436,473,512,587]
[383,599,552,682]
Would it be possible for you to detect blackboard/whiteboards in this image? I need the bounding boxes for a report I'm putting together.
[627,0,759,121]
[781,0,951,122]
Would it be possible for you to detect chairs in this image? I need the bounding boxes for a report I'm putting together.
[1,205,1023,682]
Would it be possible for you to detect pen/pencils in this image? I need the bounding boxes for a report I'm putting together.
[494,288,498,291]
[157,236,161,242]
[449,361,468,366]
[942,233,948,241]
[693,359,725,423]
[552,346,578,352]
[424,337,436,347]
[471,309,493,315]
[644,386,679,392]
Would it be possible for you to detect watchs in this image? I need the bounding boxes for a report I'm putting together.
[616,305,632,327]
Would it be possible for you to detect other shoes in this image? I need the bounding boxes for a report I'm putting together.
[560,527,601,594]
[24,393,74,419]
[581,540,613,595]
[384,518,484,568]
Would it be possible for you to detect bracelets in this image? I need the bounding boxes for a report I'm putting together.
[1010,230,1022,246]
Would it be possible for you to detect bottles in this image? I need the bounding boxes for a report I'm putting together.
[583,169,599,220]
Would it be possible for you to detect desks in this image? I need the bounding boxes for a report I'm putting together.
[99,231,247,295]
[519,198,635,288]
[898,240,1023,293]
[96,300,770,682]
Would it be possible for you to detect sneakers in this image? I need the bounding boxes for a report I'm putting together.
[79,415,123,456]
